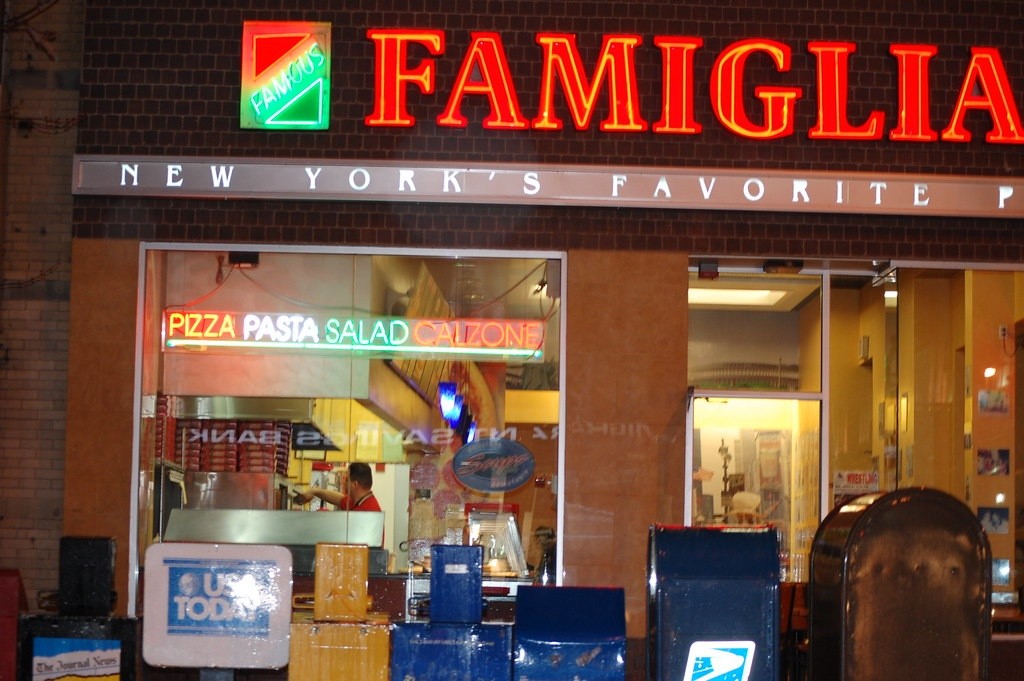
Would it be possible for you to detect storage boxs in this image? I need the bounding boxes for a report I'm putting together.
[155,393,291,475]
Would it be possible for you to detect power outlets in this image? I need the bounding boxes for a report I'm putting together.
[998,325,1007,340]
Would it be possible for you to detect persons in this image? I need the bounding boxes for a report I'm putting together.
[991,513,1008,531]
[977,453,1007,476]
[529,526,557,584]
[980,511,993,533]
[295,461,385,549]
[487,534,497,560]
